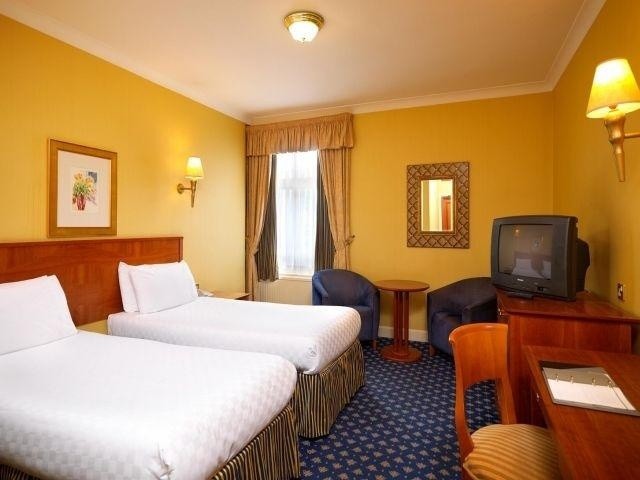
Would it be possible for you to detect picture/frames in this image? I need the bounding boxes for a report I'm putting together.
[45,139,117,239]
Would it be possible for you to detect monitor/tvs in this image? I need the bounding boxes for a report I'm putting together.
[490,215,590,300]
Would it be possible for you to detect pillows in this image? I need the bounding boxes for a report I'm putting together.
[129,260,199,315]
[0,272,79,361]
[118,261,179,314]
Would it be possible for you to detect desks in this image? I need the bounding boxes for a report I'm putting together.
[372,280,430,363]
[521,343,639,480]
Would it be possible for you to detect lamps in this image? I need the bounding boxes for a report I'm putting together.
[179,156,204,209]
[585,54,640,183]
[284,11,325,43]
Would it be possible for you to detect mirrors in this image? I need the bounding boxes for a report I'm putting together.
[406,161,471,249]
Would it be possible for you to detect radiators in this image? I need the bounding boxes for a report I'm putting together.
[258,276,312,305]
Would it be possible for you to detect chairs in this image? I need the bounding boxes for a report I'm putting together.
[312,268,381,351]
[427,276,498,357]
[444,321,568,480]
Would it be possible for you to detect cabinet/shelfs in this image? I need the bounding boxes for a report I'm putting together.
[494,286,640,426]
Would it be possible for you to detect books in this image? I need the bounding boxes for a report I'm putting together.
[540,358,640,417]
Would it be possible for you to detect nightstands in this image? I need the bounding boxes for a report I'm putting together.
[208,290,251,300]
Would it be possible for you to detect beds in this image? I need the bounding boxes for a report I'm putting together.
[108,237,367,438]
[2,228,305,478]
